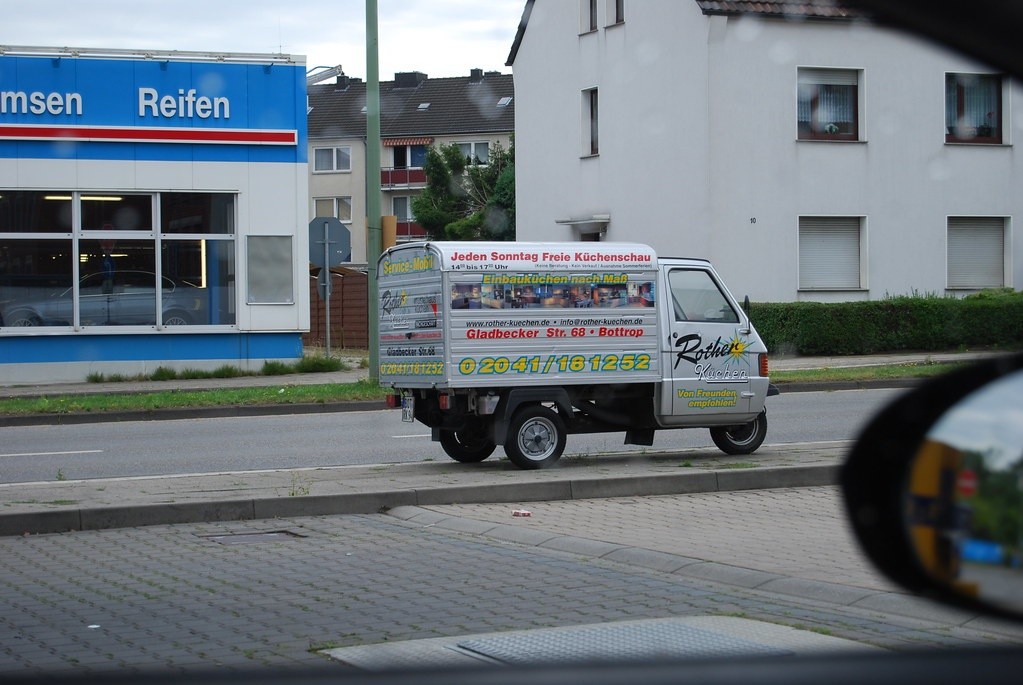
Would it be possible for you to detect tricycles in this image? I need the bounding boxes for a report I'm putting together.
[377,243,781,470]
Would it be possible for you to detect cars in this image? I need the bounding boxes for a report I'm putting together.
[0,264,222,327]
